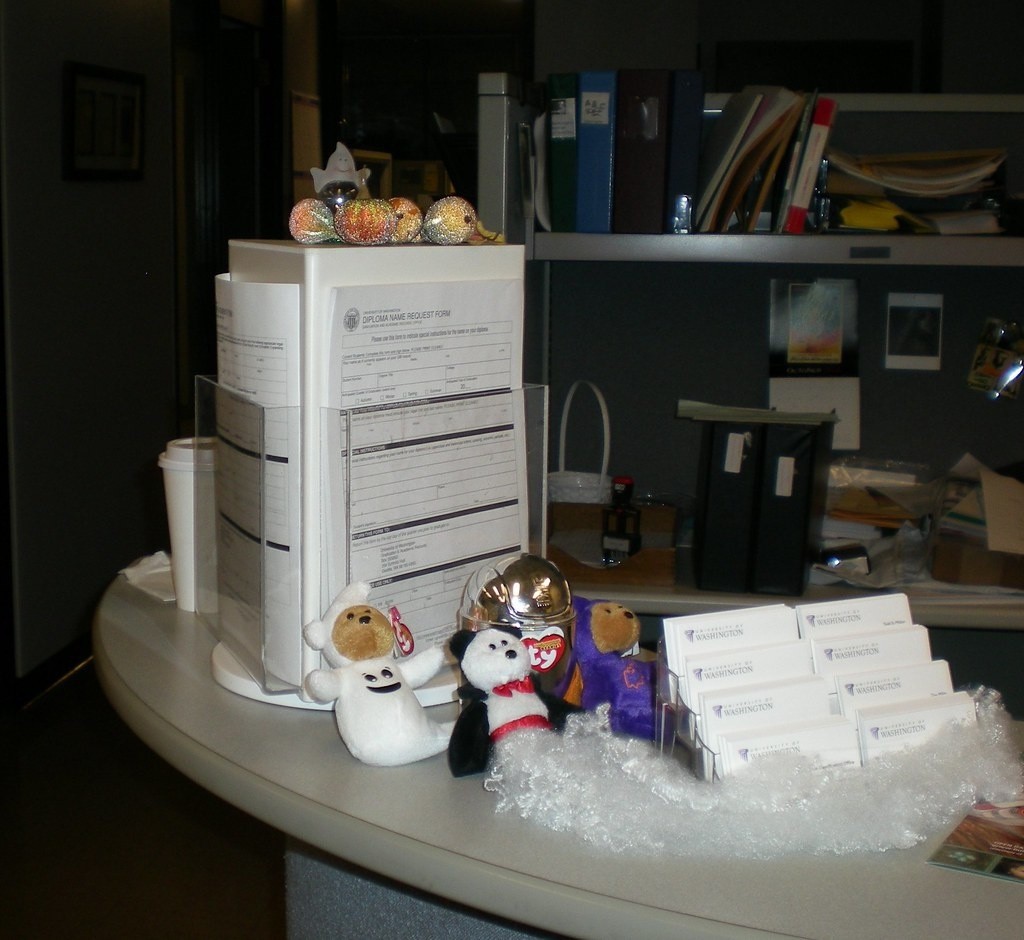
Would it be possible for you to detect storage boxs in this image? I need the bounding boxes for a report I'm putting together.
[929,454,1024,584]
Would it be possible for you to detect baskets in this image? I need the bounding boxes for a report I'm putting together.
[547,380,614,503]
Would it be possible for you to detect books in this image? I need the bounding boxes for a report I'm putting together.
[686,70,1024,597]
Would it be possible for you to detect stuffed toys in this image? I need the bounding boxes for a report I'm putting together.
[307,581,660,776]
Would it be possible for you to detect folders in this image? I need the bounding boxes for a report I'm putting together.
[548,66,706,236]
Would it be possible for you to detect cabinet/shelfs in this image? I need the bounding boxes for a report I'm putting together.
[475,72,1024,631]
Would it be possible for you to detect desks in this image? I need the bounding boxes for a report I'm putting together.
[93,558,1024,940]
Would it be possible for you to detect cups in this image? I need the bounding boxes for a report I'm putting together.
[158,437,220,613]
